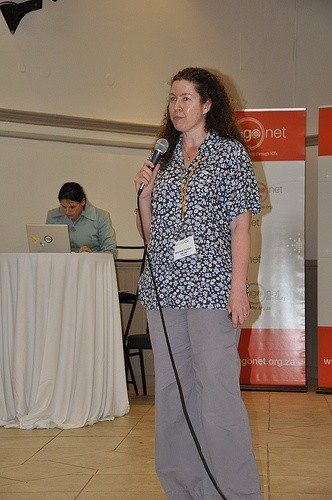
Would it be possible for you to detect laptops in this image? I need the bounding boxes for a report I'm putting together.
[26,223,71,253]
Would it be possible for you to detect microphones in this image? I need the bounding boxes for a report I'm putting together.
[138,138,169,195]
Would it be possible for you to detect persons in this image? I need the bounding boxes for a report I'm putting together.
[44,182,116,258]
[135,68,262,500]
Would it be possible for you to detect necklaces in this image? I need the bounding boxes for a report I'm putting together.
[183,142,202,160]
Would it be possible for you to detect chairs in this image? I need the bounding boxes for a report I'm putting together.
[128,322,153,397]
[114,244,152,395]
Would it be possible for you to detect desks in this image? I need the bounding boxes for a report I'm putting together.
[0,254,130,430]
